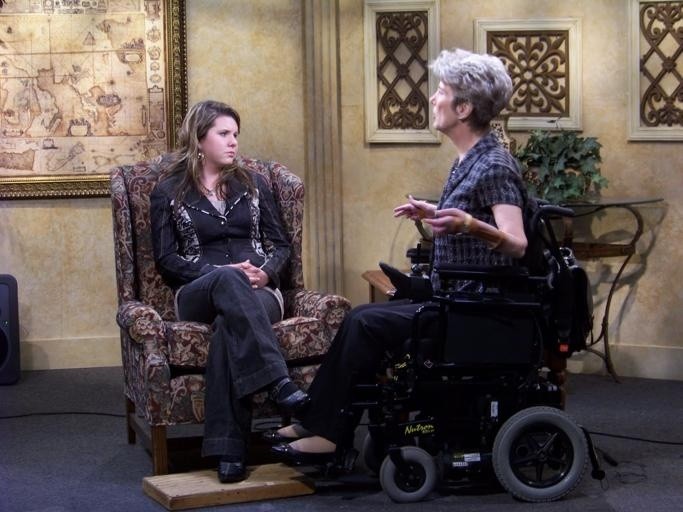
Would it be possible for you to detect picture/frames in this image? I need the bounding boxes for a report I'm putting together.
[0,0,194,203]
[626,0,683,142]
[474,18,583,131]
[362,0,443,147]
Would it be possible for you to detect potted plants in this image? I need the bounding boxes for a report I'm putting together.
[514,128,610,204]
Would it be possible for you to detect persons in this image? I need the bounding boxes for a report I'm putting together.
[264,47,529,468]
[149,100,312,483]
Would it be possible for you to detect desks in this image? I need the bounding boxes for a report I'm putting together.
[410,194,665,381]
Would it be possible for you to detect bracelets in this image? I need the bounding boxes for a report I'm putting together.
[464,212,472,235]
[487,230,504,252]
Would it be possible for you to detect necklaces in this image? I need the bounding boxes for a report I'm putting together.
[199,182,218,196]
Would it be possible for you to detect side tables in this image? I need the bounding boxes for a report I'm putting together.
[360,267,412,305]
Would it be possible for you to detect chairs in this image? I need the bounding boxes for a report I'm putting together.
[109,156,352,475]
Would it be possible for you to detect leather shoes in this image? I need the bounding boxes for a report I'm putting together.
[217,457,248,485]
[270,377,311,409]
[262,428,344,462]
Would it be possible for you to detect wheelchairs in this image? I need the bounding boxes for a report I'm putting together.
[254,198,593,503]
